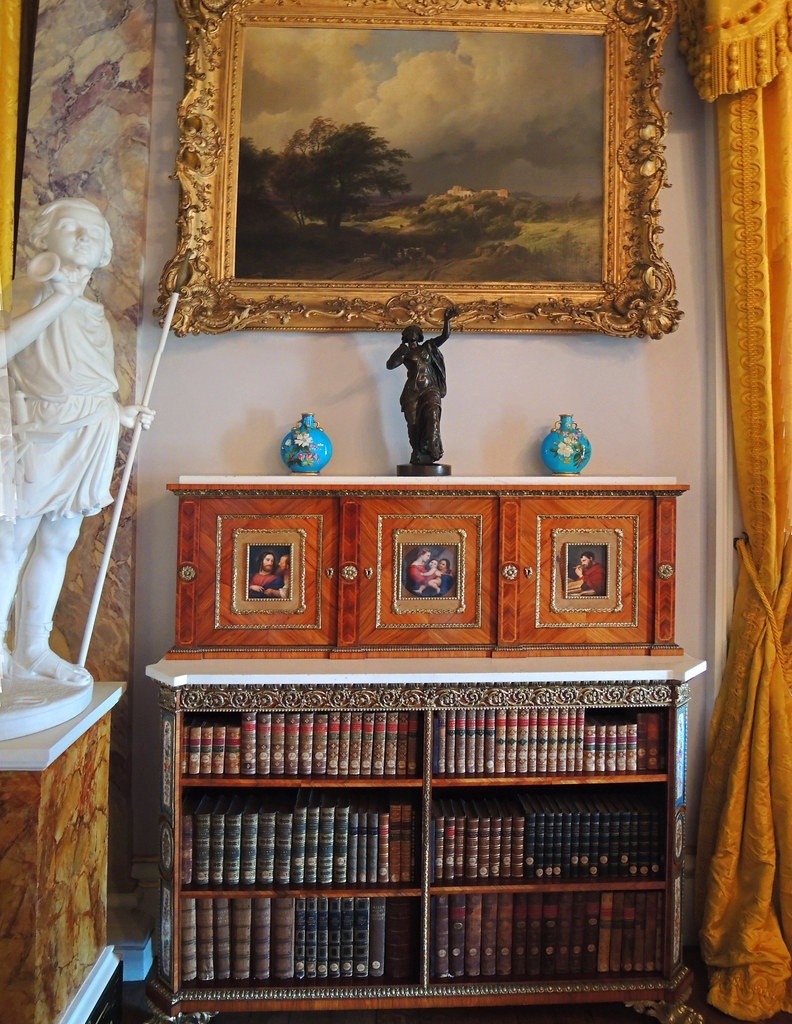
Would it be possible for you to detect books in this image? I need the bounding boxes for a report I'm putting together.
[180,706,669,986]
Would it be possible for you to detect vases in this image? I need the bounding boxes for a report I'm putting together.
[540,414,592,477]
[281,413,333,475]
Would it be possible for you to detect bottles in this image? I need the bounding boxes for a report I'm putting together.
[541,413,592,476]
[280,412,333,476]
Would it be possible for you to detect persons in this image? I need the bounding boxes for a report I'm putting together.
[0,197,157,685]
[386,306,460,464]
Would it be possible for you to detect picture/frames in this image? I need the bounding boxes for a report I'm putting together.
[151,1,684,340]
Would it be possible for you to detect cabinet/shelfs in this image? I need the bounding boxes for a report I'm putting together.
[144,475,704,1024]
